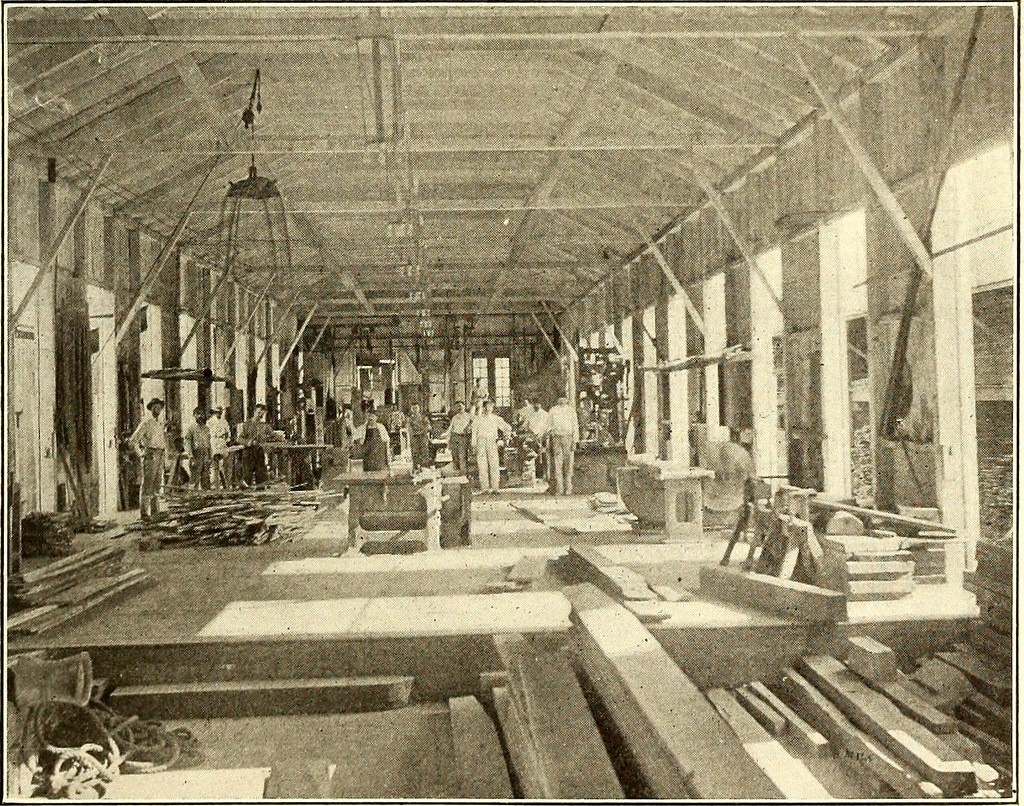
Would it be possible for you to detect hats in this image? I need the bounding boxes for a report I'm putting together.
[147,398,164,409]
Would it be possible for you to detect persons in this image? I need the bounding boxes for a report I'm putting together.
[543,392,581,495]
[407,403,434,471]
[523,398,550,478]
[184,406,214,491]
[515,395,535,425]
[343,410,395,478]
[236,404,286,493]
[470,377,488,422]
[204,405,234,491]
[470,400,512,493]
[440,400,474,478]
[130,397,168,521]
